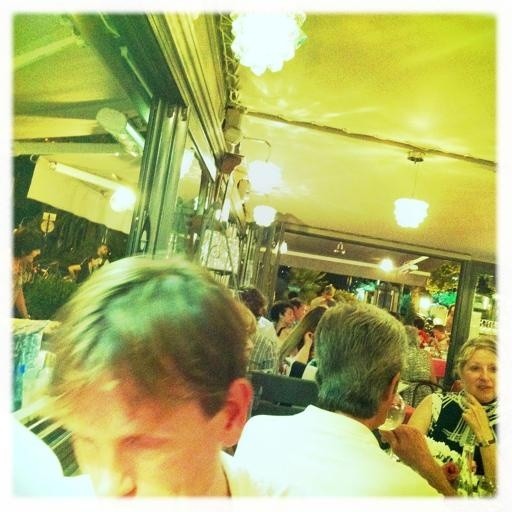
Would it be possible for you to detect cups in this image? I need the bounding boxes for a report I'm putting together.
[375,391,408,433]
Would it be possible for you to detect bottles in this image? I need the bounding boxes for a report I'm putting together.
[453,444,478,496]
